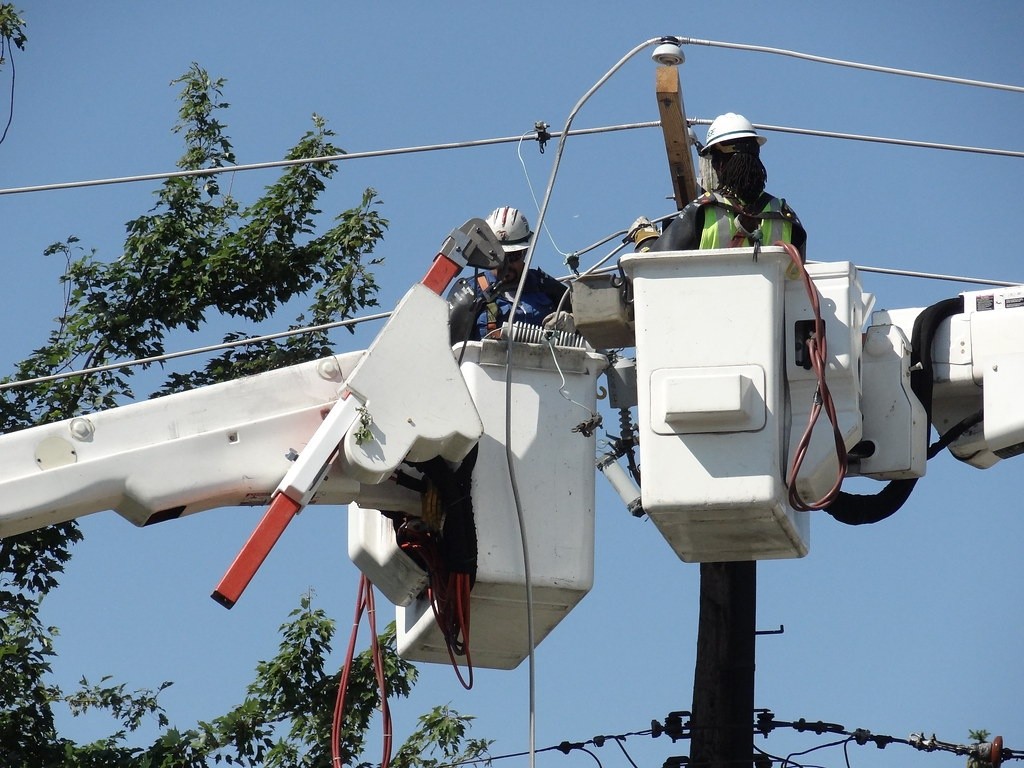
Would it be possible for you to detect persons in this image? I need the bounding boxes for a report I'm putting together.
[446,205,573,342]
[622,112,808,266]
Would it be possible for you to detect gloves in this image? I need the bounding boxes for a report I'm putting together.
[627,215,662,252]
[542,310,577,334]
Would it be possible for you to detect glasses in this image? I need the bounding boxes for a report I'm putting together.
[506,251,525,263]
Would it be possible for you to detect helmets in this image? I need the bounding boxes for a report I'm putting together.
[485,206,534,252]
[701,112,767,153]
